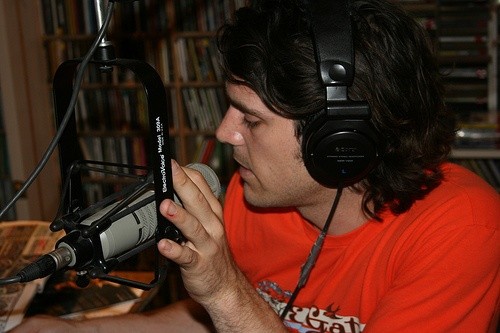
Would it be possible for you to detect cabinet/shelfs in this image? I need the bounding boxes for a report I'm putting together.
[41,0,252,237]
[388,0,500,195]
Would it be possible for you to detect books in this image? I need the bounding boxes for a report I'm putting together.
[0,221,67,332]
[57,279,145,318]
[44,0,247,205]
[391,0,500,192]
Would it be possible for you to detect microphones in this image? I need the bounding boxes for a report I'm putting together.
[17,163,221,283]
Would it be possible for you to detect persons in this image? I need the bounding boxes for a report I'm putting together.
[7,0,500,332]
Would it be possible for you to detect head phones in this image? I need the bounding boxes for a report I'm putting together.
[301,0,385,189]
